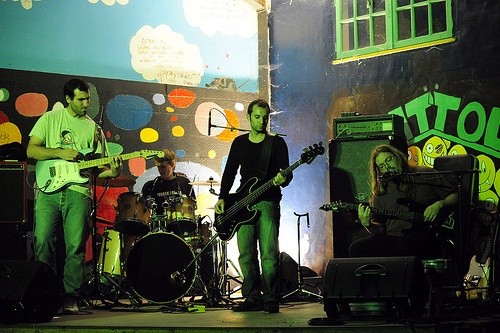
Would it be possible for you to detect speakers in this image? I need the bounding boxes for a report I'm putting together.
[329,137,406,258]
[435,156,480,205]
[0,161,28,222]
[0,259,66,324]
[322,257,431,326]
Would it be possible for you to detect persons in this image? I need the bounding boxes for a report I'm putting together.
[349,144,460,258]
[214,99,293,313]
[144,148,197,216]
[26,78,123,316]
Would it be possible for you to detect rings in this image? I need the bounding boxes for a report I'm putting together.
[275,181,279,184]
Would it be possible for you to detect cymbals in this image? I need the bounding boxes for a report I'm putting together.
[188,180,222,187]
[92,216,114,224]
[90,173,137,187]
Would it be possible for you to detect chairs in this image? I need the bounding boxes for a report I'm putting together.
[419,155,480,308]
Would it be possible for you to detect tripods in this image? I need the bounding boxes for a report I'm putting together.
[65,127,325,313]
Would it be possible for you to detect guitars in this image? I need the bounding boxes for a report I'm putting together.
[318,197,459,236]
[215,141,325,241]
[35,147,166,195]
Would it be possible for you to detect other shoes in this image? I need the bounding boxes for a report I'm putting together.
[234,299,262,312]
[263,301,279,313]
[62,302,79,316]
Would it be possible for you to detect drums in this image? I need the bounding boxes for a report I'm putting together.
[183,224,210,246]
[114,192,153,236]
[122,230,198,304]
[165,197,197,234]
